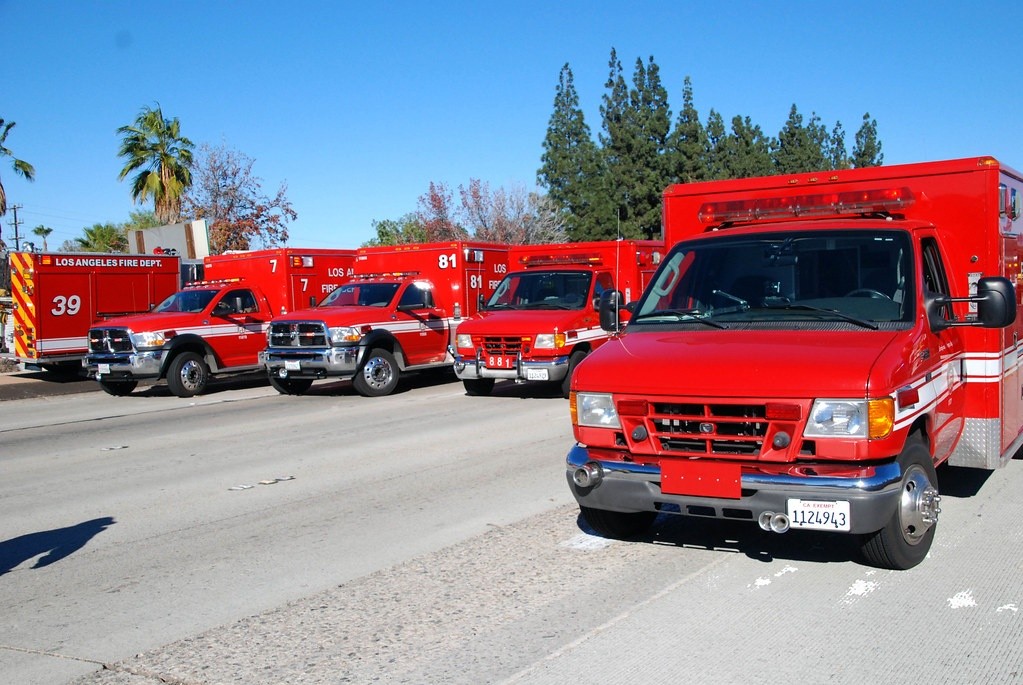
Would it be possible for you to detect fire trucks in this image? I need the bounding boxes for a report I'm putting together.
[84,248,358,399]
[0,250,204,383]
[566,154,1022,570]
[454,241,665,398]
[256,241,514,397]
